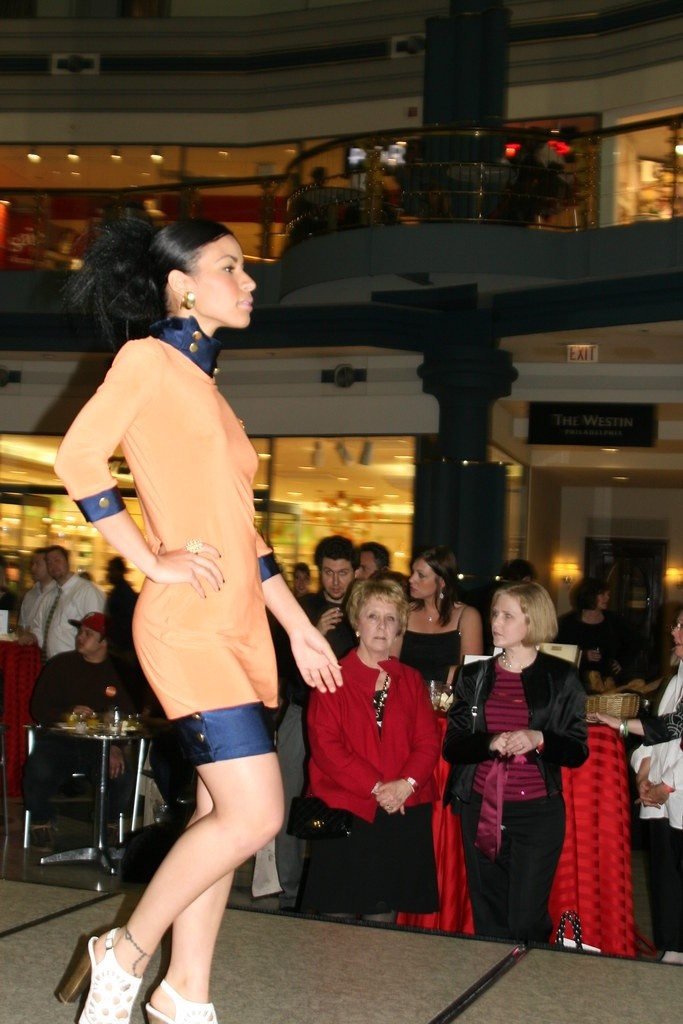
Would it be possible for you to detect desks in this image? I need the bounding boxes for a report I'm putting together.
[395,704,638,957]
[38,717,171,874]
[0,641,42,797]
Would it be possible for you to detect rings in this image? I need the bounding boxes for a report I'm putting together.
[187,537,202,553]
[387,805,392,809]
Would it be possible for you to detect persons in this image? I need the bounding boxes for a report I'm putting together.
[0,534,683,968]
[53,212,344,1024]
[398,139,449,216]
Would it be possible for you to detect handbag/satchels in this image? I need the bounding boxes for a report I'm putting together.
[287,796,352,841]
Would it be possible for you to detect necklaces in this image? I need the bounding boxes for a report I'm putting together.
[423,607,437,622]
[502,651,533,668]
[373,674,389,717]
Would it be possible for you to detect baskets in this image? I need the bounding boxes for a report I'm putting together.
[586,694,640,721]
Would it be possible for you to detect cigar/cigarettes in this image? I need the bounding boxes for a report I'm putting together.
[335,608,339,623]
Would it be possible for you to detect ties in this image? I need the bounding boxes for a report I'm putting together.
[41,589,62,664]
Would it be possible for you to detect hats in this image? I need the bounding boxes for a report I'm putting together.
[68,612,106,635]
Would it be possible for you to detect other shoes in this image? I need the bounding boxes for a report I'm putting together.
[30,827,53,851]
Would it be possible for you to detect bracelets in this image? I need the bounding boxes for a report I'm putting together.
[618,719,624,737]
[402,776,418,793]
[625,718,629,736]
[661,781,675,792]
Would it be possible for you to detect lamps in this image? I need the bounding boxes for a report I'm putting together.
[335,442,356,465]
[361,441,373,466]
[311,442,324,466]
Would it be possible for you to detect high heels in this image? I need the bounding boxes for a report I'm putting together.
[146,979,218,1024]
[57,927,144,1024]
